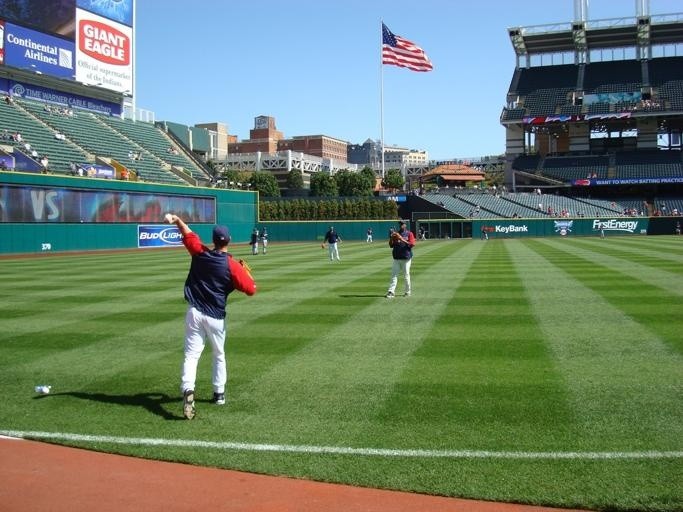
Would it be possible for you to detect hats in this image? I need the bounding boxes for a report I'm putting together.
[213,226,230,242]
[398,220,407,224]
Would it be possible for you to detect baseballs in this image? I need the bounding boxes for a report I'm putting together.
[164,213,173,222]
[40,385,50,396]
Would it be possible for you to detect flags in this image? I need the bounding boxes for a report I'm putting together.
[380,22,432,72]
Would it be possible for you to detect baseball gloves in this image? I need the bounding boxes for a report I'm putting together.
[321,242,325,248]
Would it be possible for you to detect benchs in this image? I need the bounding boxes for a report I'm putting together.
[1,94,214,190]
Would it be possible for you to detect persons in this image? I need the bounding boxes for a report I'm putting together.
[627,106,632,111]
[163,212,256,421]
[127,151,132,158]
[633,105,636,111]
[675,220,681,236]
[621,107,625,113]
[388,226,393,235]
[419,226,426,240]
[411,184,682,218]
[133,152,138,160]
[599,223,605,238]
[384,220,415,299]
[323,226,342,262]
[616,108,621,113]
[248,227,259,255]
[259,227,267,254]
[165,145,178,156]
[1,94,73,170]
[366,228,372,242]
[591,171,597,179]
[482,230,488,240]
[138,153,143,161]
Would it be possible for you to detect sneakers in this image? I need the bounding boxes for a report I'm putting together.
[183,387,196,420]
[403,292,412,297]
[213,392,225,405]
[385,291,395,298]
